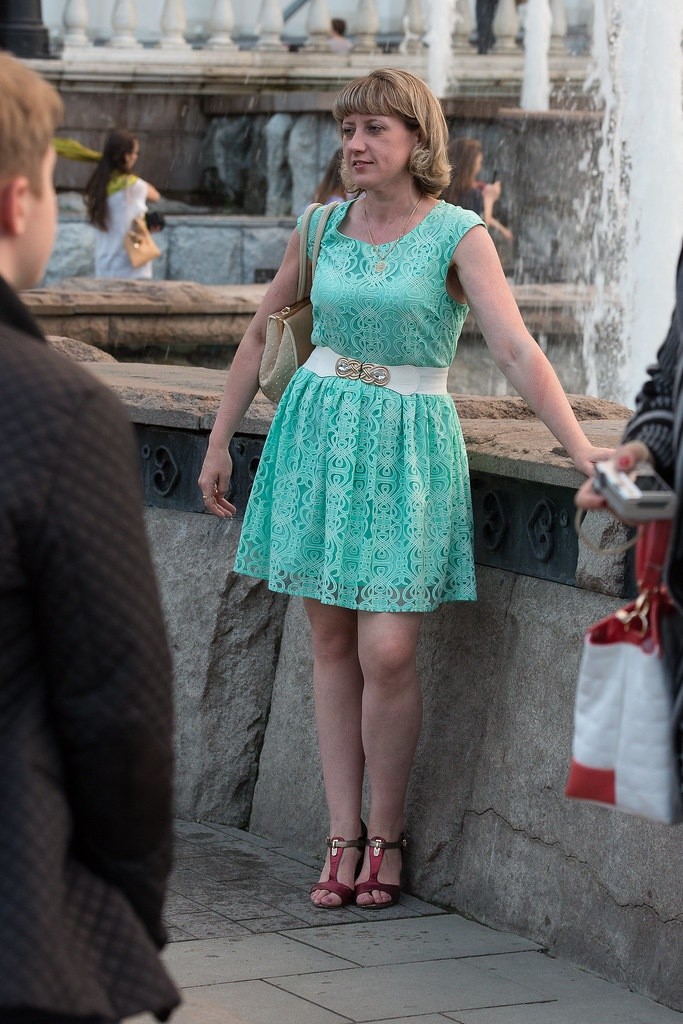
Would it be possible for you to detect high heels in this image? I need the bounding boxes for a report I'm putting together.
[354,830,406,908]
[308,819,367,908]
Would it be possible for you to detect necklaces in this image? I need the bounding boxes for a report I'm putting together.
[362,194,424,273]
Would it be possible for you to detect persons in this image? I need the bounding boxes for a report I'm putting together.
[82,124,165,280]
[197,70,615,912]
[571,232,683,607]
[312,144,353,210]
[475,1,498,57]
[326,19,356,54]
[447,137,516,242]
[0,48,181,1024]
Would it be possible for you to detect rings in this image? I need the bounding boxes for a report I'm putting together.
[202,493,214,500]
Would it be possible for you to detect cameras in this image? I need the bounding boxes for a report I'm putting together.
[593,457,678,523]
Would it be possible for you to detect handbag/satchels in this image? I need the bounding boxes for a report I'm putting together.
[562,520,681,825]
[125,217,160,267]
[258,201,340,403]
